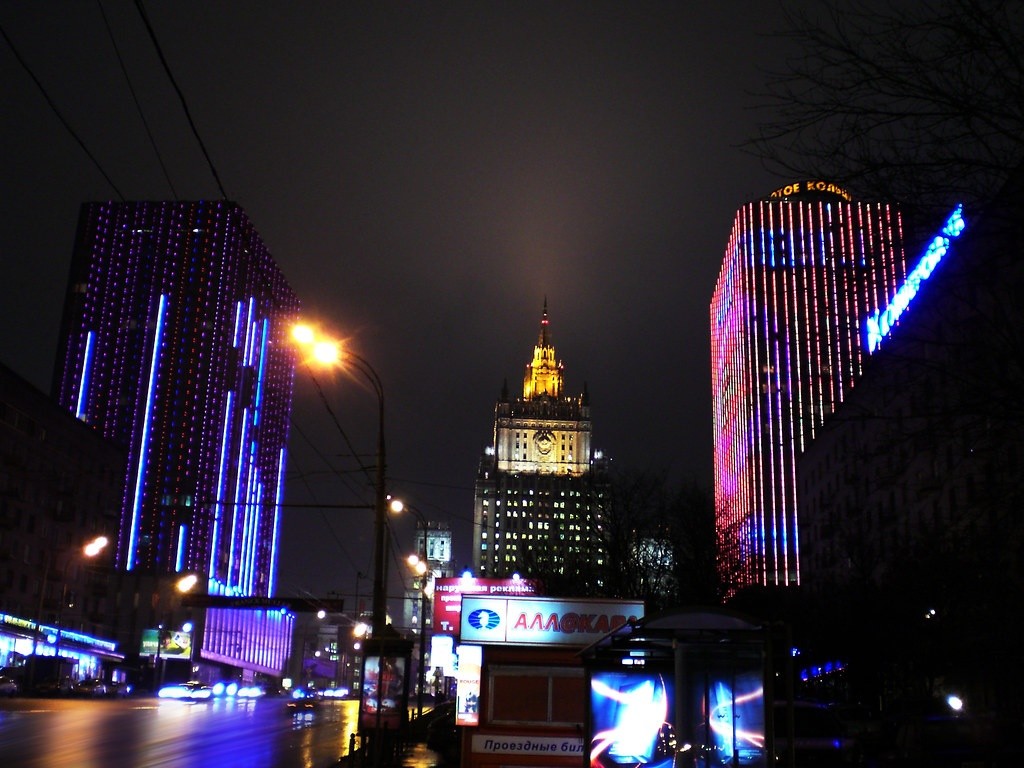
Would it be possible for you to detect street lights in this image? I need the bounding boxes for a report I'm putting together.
[55,536,109,659]
[390,497,430,722]
[290,321,390,641]
[152,574,198,664]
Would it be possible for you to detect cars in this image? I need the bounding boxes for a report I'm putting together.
[0,665,324,718]
